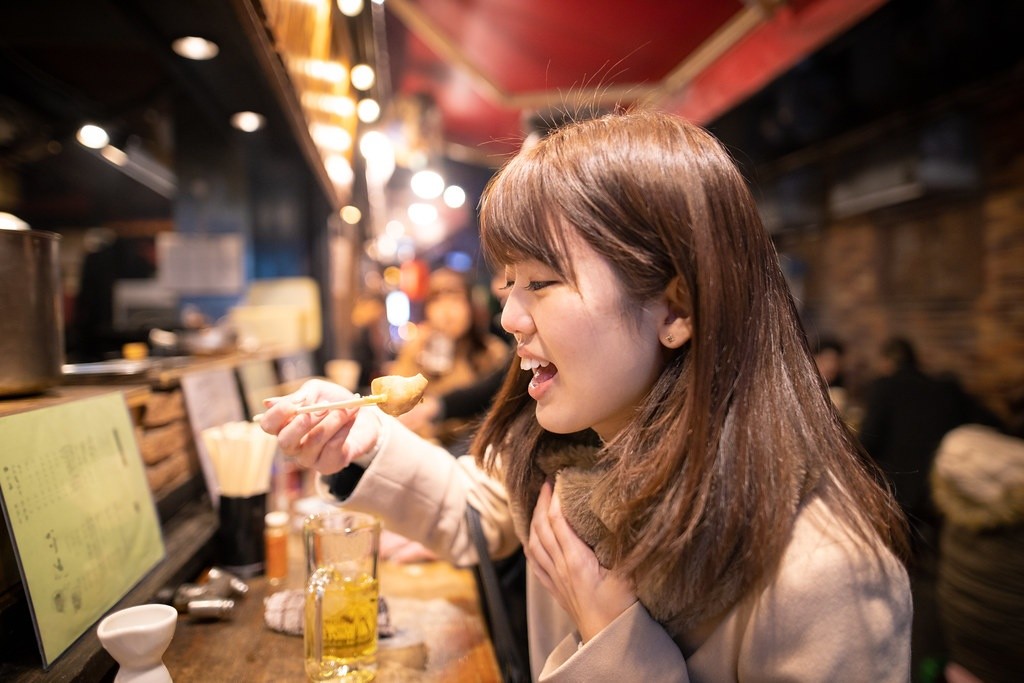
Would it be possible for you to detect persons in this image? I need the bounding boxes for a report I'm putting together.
[467,103,649,683]
[796,318,1024,683]
[323,226,552,468]
[257,109,917,683]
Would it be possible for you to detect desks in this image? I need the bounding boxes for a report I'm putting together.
[150,558,509,683]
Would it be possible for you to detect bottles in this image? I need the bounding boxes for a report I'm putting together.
[96,602,179,683]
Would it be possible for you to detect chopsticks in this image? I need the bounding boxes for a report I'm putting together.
[253,394,388,432]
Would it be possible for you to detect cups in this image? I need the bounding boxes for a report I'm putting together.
[303,511,381,683]
